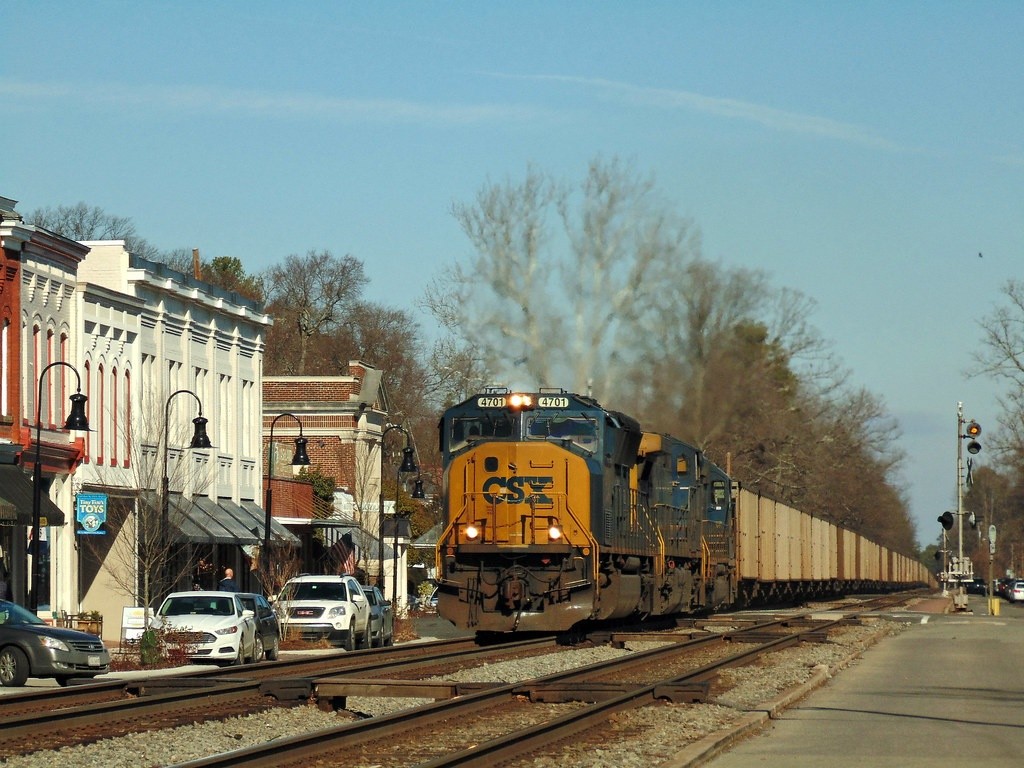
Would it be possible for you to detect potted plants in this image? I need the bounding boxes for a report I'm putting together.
[77,611,90,631]
[88,610,103,634]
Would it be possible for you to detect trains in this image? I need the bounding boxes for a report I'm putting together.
[436,387,939,633]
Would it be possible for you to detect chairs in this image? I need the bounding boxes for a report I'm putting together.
[175,603,194,614]
[366,594,374,606]
[216,600,230,615]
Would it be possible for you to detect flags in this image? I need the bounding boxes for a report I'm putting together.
[315,530,355,575]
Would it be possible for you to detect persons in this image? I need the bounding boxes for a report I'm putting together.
[216,569,240,592]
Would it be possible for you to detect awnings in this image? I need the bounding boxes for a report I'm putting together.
[335,525,400,560]
[0,465,65,528]
[137,492,302,546]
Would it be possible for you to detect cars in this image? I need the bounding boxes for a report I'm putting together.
[0,600,111,686]
[237,592,280,663]
[971,577,1024,603]
[359,585,393,647]
[149,591,256,665]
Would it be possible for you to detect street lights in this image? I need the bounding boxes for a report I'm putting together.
[378,423,416,598]
[25,361,90,618]
[259,412,312,593]
[392,463,425,619]
[158,389,212,603]
[936,400,982,615]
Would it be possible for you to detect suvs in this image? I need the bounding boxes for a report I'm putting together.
[266,573,373,652]
